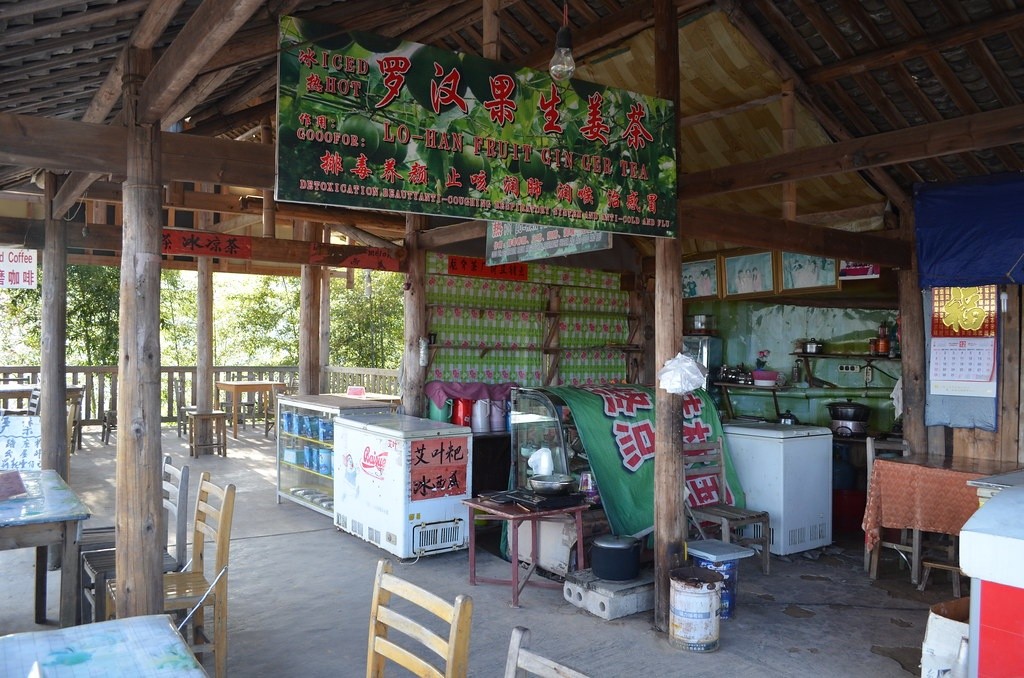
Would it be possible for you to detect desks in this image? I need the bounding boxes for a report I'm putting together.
[461,498,590,609]
[0,384,86,407]
[861,452,1024,584]
[0,468,94,629]
[215,379,286,439]
[0,614,212,678]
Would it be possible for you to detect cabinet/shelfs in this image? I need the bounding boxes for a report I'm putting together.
[425,273,648,386]
[275,393,397,519]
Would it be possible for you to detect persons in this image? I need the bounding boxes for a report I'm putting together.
[820,258,827,286]
[792,258,819,288]
[682,269,711,298]
[827,261,835,286]
[736,267,762,292]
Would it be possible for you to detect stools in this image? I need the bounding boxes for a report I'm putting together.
[187,409,227,459]
[917,561,960,598]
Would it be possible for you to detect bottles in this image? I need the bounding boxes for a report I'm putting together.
[717,363,753,384]
[869,319,900,358]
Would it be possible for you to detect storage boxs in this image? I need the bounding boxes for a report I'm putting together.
[919,596,969,678]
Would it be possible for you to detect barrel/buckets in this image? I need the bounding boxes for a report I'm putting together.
[691,558,739,621]
[666,566,724,654]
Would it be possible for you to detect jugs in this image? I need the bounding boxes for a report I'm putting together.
[428,384,511,433]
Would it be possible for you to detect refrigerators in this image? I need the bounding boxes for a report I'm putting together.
[721,422,833,558]
[333,411,474,558]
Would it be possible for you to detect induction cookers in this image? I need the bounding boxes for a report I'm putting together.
[506,488,583,511]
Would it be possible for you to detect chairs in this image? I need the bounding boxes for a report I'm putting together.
[172,375,198,438]
[683,437,771,576]
[220,373,258,430]
[863,437,910,572]
[80,455,590,678]
[264,384,299,438]
[0,376,117,454]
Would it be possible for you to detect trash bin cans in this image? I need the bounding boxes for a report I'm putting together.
[921,596,970,678]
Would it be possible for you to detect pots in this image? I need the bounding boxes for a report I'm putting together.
[800,338,823,353]
[527,474,575,494]
[777,410,797,425]
[824,398,875,422]
[590,528,642,580]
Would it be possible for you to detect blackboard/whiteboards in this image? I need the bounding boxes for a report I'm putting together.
[0,416,41,470]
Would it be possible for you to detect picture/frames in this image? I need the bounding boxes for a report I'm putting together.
[681,243,842,303]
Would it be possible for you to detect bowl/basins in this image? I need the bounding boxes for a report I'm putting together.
[685,313,722,330]
[752,370,778,386]
